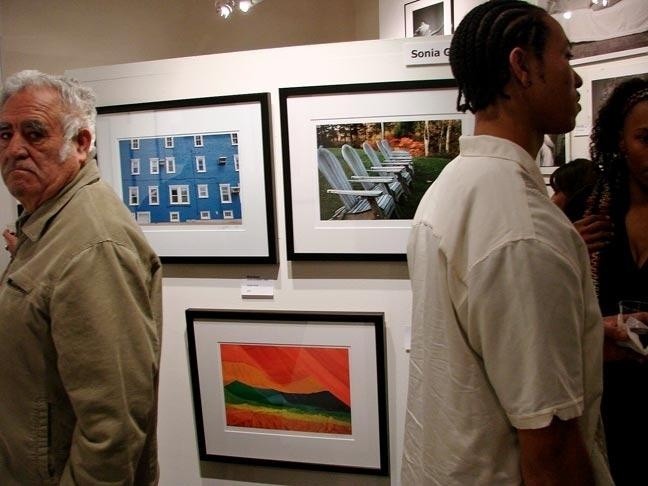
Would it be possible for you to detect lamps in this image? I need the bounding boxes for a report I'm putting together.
[219,1,236,16]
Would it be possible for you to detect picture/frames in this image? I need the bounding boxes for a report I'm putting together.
[279,79,470,261]
[185,308,390,477]
[403,0,454,37]
[95,92,279,267]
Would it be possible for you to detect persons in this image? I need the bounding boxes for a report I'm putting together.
[0,69,164,486]
[398,0,646,485]
[543,157,599,218]
[568,76,647,484]
